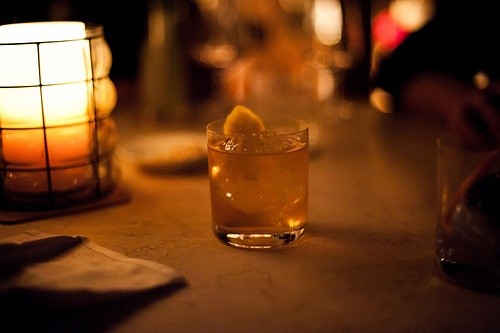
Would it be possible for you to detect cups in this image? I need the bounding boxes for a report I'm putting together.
[435,134,500,277]
[206,116,310,249]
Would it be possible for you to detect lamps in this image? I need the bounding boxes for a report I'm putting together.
[0,21,132,226]
